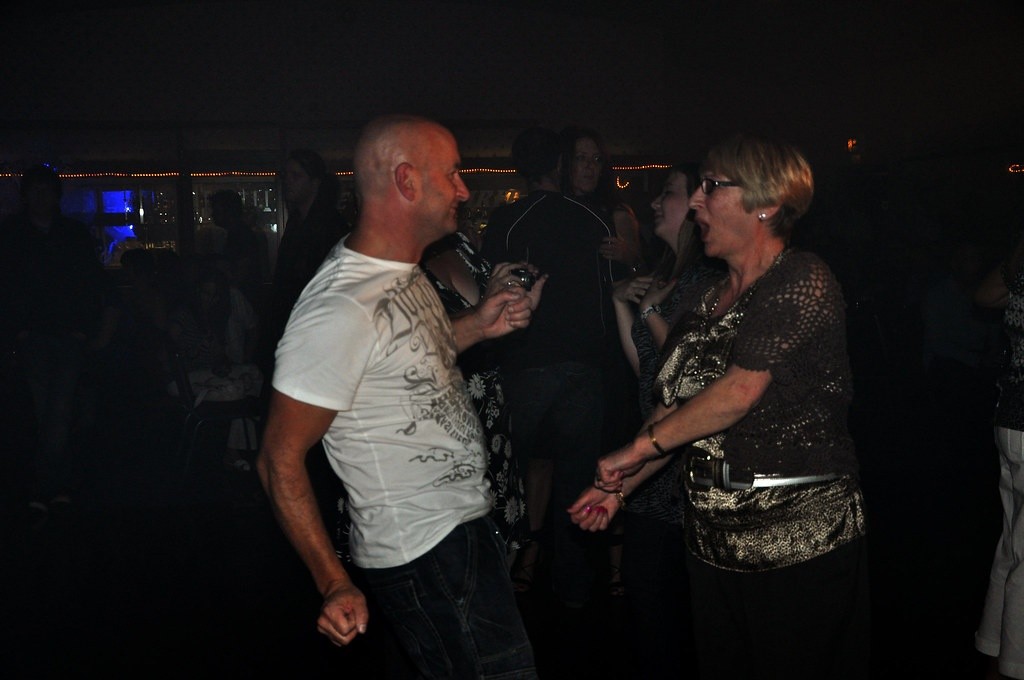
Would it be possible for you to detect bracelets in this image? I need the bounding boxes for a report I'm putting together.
[616,490,627,511]
[641,305,662,327]
[647,424,667,456]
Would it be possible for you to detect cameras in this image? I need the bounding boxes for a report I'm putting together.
[508,268,536,293]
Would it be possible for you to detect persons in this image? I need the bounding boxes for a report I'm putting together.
[0,127,1024,680]
[253,117,535,680]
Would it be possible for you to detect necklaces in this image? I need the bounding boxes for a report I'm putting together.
[696,246,796,389]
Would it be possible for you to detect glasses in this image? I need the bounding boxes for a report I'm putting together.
[697,177,742,194]
